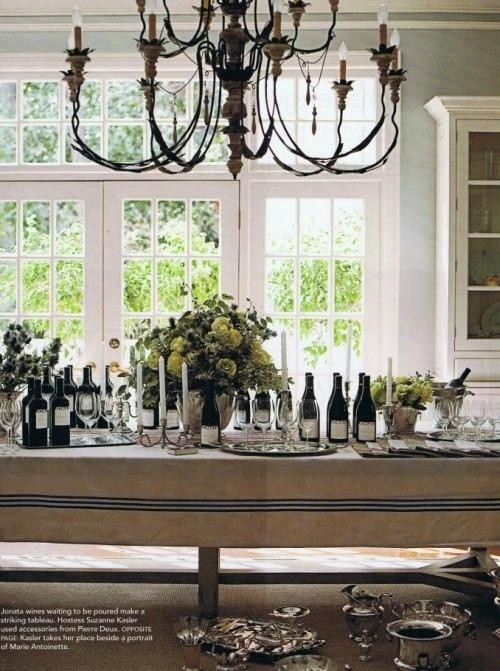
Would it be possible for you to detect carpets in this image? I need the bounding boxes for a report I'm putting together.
[1,583,500,671]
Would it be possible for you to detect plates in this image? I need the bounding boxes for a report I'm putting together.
[15,432,137,450]
[218,440,335,457]
[426,427,499,442]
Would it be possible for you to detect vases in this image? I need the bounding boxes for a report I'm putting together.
[394,408,419,435]
[175,389,236,434]
[1,392,22,430]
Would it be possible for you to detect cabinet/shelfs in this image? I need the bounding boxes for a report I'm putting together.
[423,96,500,419]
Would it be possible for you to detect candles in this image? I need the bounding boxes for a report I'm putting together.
[182,361,190,424]
[159,356,166,419]
[137,362,143,426]
[386,355,393,405]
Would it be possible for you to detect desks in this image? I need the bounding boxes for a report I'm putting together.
[1,430,499,620]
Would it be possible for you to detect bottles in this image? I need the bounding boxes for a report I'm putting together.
[253,388,270,430]
[77,366,97,429]
[98,365,115,427]
[353,373,376,442]
[444,367,471,388]
[234,388,250,429]
[22,367,53,446]
[300,373,320,441]
[327,373,348,442]
[201,381,220,447]
[49,365,77,445]
[276,388,292,429]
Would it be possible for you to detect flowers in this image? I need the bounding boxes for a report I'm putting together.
[116,283,295,411]
[370,370,435,410]
[0,323,62,391]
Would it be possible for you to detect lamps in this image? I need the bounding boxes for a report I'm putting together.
[61,0,407,181]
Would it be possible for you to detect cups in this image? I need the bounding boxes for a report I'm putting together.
[0,398,11,454]
[486,400,499,440]
[473,412,487,439]
[8,398,23,451]
[439,400,455,439]
[296,400,319,451]
[75,391,97,444]
[234,398,259,450]
[450,401,463,440]
[453,400,471,440]
[100,390,119,441]
[86,394,102,444]
[276,398,287,442]
[3,400,13,451]
[281,401,299,452]
[434,403,445,438]
[251,397,277,451]
[110,401,129,439]
[469,400,488,440]
[488,420,499,435]
